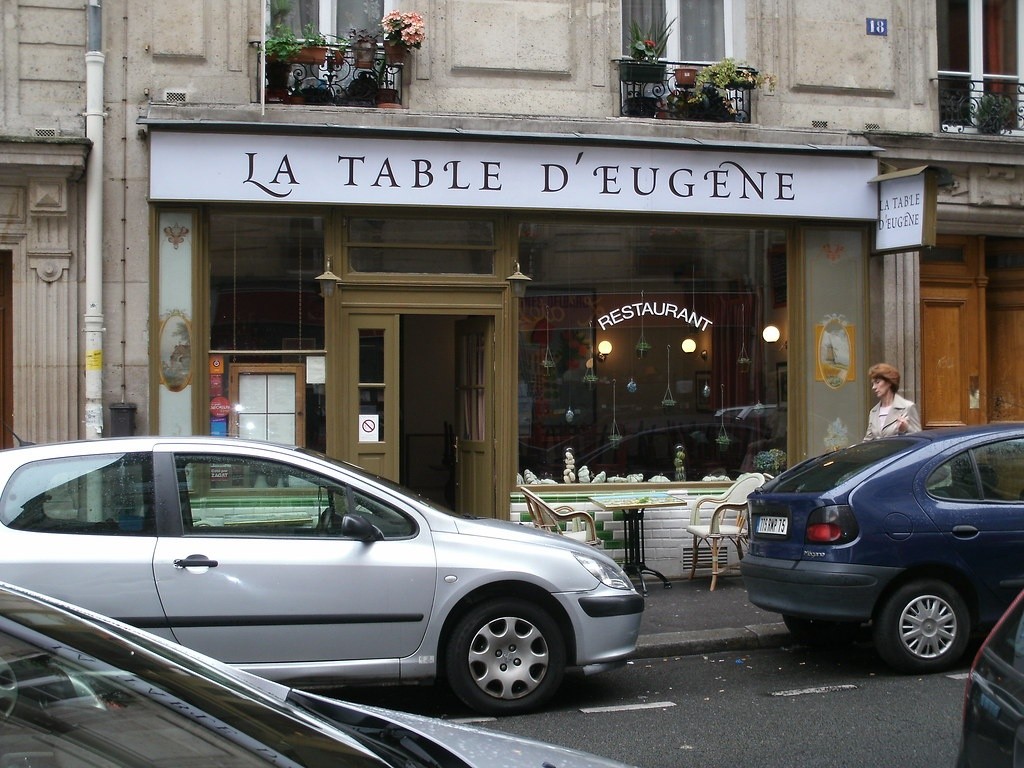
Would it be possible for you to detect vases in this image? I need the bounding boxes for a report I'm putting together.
[376,88,398,103]
[384,40,410,65]
[618,62,667,84]
[672,68,699,86]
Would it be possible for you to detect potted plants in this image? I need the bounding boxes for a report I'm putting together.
[703,59,774,92]
[979,94,1013,135]
[663,400,676,417]
[582,375,599,391]
[265,20,329,64]
[633,343,651,359]
[609,434,623,449]
[736,358,752,374]
[539,361,556,377]
[289,80,304,104]
[715,435,732,452]
[349,28,378,68]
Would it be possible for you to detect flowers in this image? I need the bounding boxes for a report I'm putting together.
[624,15,676,64]
[382,9,426,51]
[752,449,786,473]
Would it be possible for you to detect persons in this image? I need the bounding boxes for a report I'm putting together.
[862,363,921,443]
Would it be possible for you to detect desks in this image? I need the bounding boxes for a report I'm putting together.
[587,492,687,597]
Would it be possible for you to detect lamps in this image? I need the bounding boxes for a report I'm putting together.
[763,325,788,352]
[505,259,532,299]
[595,341,613,363]
[315,258,343,297]
[681,338,707,361]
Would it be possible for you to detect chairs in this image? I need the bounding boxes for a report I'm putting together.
[687,473,766,592]
[520,486,601,549]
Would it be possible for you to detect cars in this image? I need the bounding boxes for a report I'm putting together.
[535,413,775,482]
[1,582,637,768]
[738,422,1024,673]
[717,404,782,423]
[948,587,1024,768]
[1,437,644,715]
